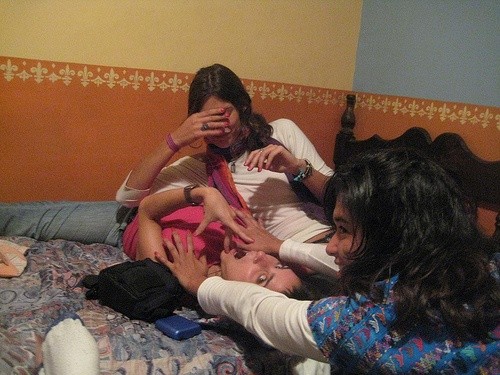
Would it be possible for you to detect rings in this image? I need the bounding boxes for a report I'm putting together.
[203,123,209,129]
[260,151,263,153]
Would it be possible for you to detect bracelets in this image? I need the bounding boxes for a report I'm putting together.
[292,160,312,181]
[166,133,182,152]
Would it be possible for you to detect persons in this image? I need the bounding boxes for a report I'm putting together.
[154,148,500,375]
[116,64,335,243]
[0,187,323,300]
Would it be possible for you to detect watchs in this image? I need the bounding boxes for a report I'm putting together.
[185,184,200,205]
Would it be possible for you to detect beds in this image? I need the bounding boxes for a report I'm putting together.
[0,94,500,375]
